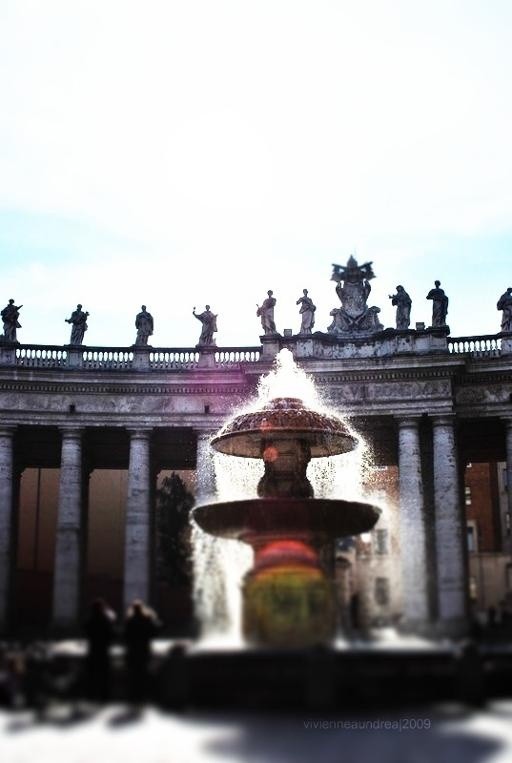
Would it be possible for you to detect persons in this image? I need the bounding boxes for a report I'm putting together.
[426,279,448,326]
[496,287,511,331]
[389,284,413,329]
[294,289,316,334]
[64,303,89,343]
[468,597,511,644]
[257,289,277,334]
[81,597,122,702]
[0,298,23,342]
[134,304,154,345]
[121,600,163,708]
[332,253,373,322]
[193,305,220,346]
